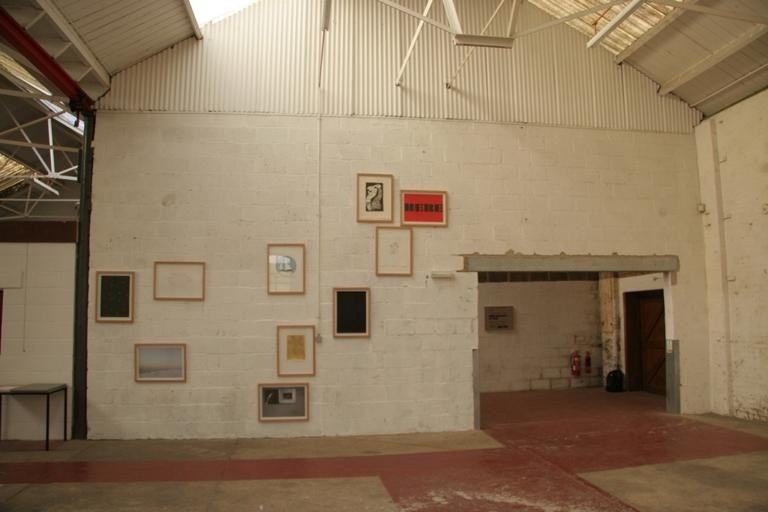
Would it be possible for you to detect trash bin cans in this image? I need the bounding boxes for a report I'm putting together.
[607,371,623,392]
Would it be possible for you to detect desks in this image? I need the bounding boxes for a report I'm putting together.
[0,382,67,451]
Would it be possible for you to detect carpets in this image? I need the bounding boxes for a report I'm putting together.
[573,447,768,512]
[0,429,509,464]
[1,473,402,511]
[683,409,768,441]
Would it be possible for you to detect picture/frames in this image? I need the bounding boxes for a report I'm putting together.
[330,286,372,338]
[257,382,310,422]
[375,226,413,277]
[266,242,306,295]
[276,323,316,377]
[355,171,394,224]
[399,189,448,228]
[95,269,135,324]
[152,260,206,302]
[134,342,187,384]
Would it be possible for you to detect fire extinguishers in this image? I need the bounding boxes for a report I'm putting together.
[570,351,580,374]
